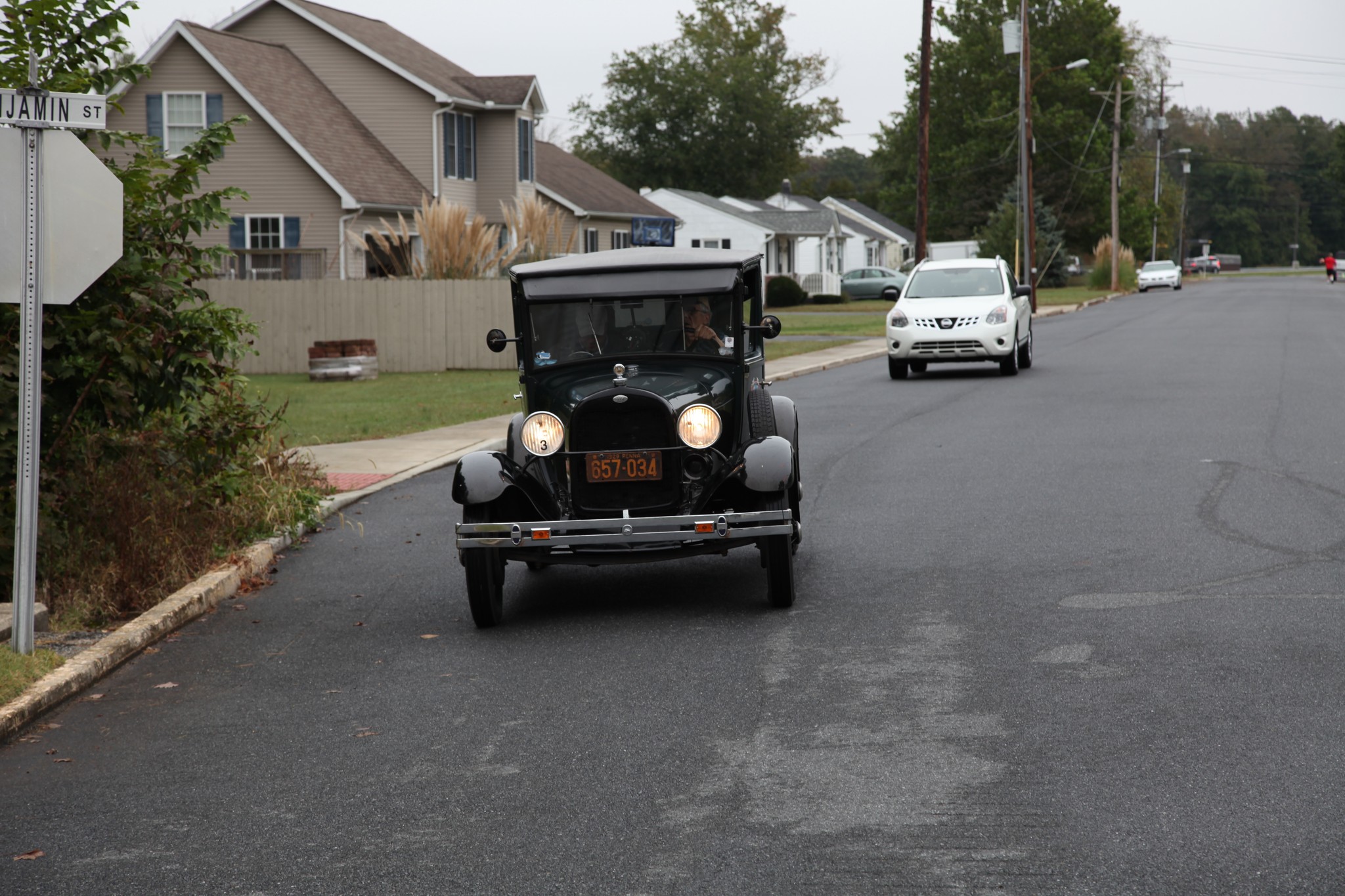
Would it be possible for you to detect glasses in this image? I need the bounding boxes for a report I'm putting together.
[684,306,709,315]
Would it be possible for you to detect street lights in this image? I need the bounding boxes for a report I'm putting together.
[1025,57,1091,318]
[1150,148,1192,261]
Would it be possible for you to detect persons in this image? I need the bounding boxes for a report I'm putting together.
[657,296,732,358]
[1325,253,1338,284]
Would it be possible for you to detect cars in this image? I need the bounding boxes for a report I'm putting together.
[449,246,803,631]
[840,265,909,301]
[1135,259,1182,293]
[885,253,1033,381]
[1182,254,1221,274]
